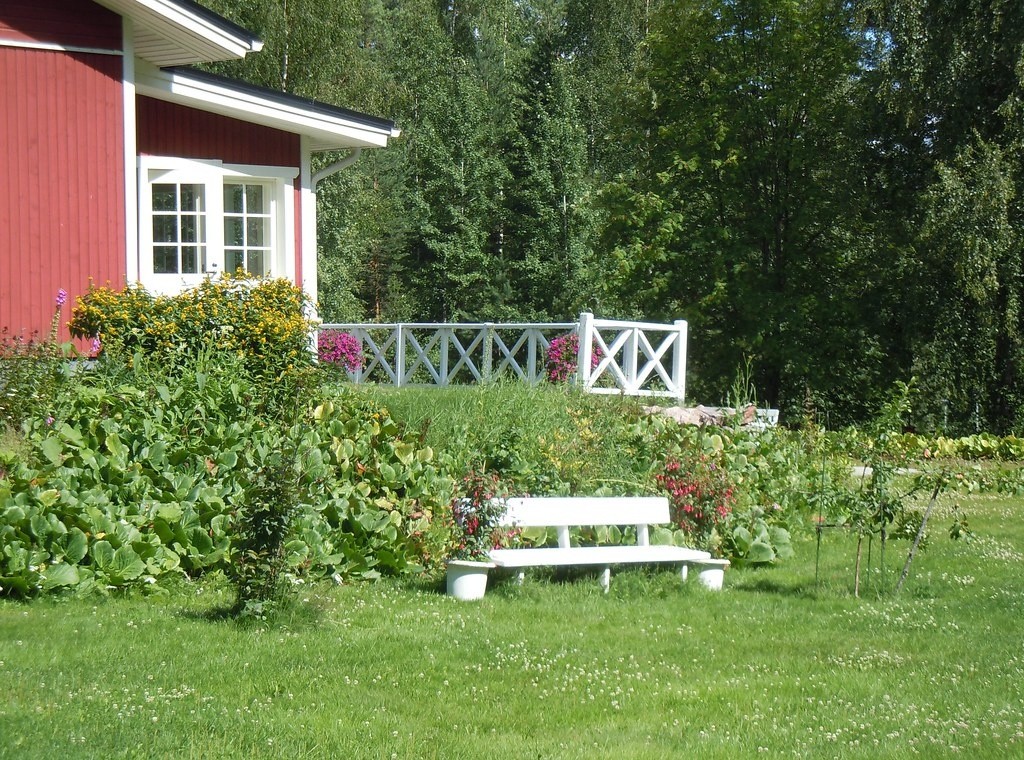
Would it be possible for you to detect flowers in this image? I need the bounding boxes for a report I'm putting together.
[656,455,739,549]
[546,332,602,383]
[318,330,365,373]
[447,472,524,562]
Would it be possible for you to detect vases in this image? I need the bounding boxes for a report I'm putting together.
[446,558,496,601]
[699,558,730,590]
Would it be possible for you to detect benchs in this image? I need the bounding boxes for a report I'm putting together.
[458,496,711,594]
